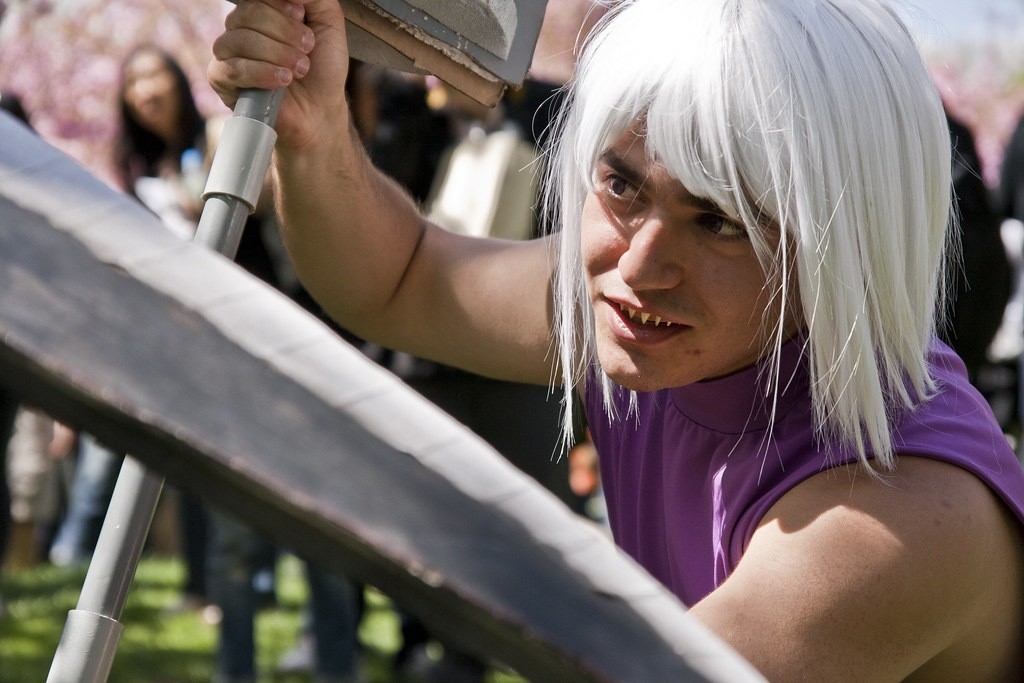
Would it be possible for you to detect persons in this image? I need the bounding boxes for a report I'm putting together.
[98,44,297,611]
[0,92,124,570]
[388,72,573,681]
[208,1,1023,682]
[934,105,1015,385]
[212,59,375,683]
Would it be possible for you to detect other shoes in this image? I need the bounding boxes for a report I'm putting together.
[276,648,317,674]
